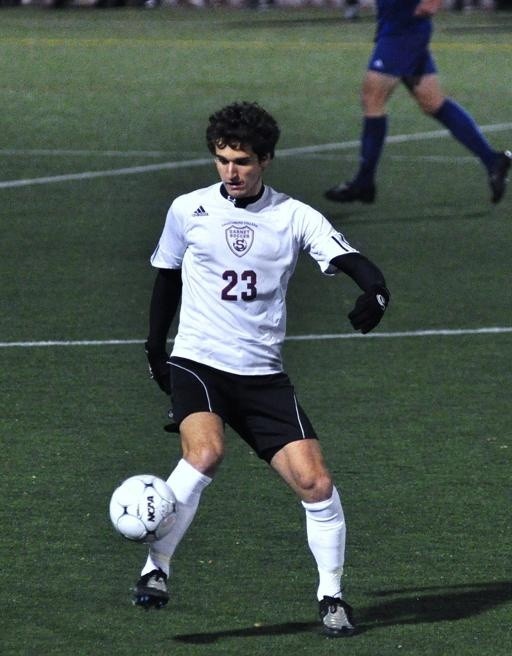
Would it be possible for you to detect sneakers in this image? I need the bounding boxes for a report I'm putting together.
[324,175,376,204]
[489,149,512,204]
[318,595,356,638]
[132,567,170,610]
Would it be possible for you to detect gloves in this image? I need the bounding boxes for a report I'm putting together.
[347,286,391,336]
[145,342,172,397]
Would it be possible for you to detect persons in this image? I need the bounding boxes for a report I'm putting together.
[129,99,390,636]
[318,0,511,210]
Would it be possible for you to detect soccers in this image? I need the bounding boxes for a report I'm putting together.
[107,474,180,544]
